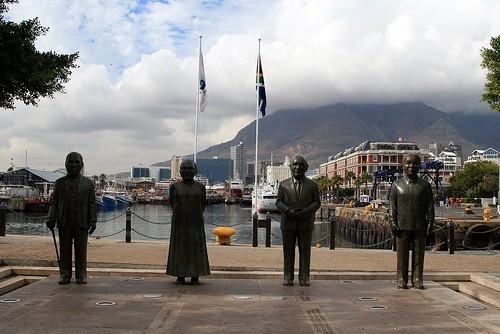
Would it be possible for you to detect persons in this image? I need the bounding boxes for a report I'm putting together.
[165,158,211,285]
[275,155,321,286]
[444,196,461,207]
[45,151,98,284]
[389,152,435,289]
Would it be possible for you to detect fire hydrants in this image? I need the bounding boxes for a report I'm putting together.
[455,197,461,207]
[449,198,454,207]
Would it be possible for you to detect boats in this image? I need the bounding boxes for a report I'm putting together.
[224,194,236,204]
[252,185,281,214]
[95,181,137,210]
[25,197,49,212]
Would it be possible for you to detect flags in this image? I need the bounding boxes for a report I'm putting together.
[255,54,266,117]
[199,47,208,112]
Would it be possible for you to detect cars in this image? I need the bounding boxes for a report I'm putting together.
[322,194,336,201]
[465,198,482,208]
